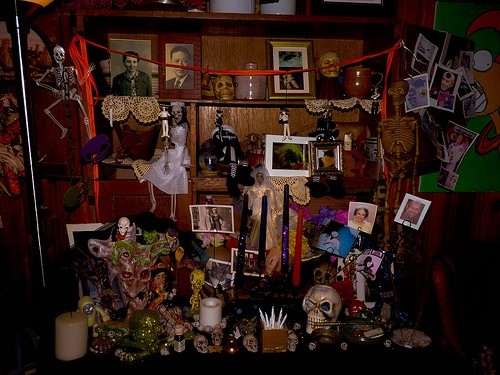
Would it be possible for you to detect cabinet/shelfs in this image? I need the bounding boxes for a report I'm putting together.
[74,9,408,232]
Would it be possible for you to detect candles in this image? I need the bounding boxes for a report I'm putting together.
[281,181,289,273]
[55,310,87,361]
[199,297,222,328]
[258,193,267,267]
[292,205,302,286]
[234,191,249,285]
[259,306,288,329]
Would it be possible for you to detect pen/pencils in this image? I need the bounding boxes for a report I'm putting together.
[259,305,288,329]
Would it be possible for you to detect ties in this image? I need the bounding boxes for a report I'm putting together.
[175,80,181,89]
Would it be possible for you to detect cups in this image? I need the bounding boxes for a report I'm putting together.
[260,318,288,352]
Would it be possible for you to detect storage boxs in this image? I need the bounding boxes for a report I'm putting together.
[259,317,287,353]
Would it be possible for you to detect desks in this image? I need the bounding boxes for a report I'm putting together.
[36,296,467,375]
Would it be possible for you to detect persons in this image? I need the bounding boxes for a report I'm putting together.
[317,231,340,255]
[214,110,223,141]
[279,108,293,140]
[166,46,194,90]
[247,167,283,251]
[202,208,224,231]
[428,71,469,189]
[113,51,153,97]
[317,50,341,77]
[399,200,425,225]
[349,206,373,233]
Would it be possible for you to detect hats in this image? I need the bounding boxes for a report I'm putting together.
[212,208,216,211]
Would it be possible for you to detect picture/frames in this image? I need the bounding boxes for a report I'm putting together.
[265,134,317,177]
[266,39,316,100]
[309,140,344,177]
[157,31,202,100]
[66,223,107,298]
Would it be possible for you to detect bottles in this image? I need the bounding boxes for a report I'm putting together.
[234,62,267,100]
[174,325,185,353]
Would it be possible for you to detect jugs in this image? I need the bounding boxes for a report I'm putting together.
[343,66,383,99]
